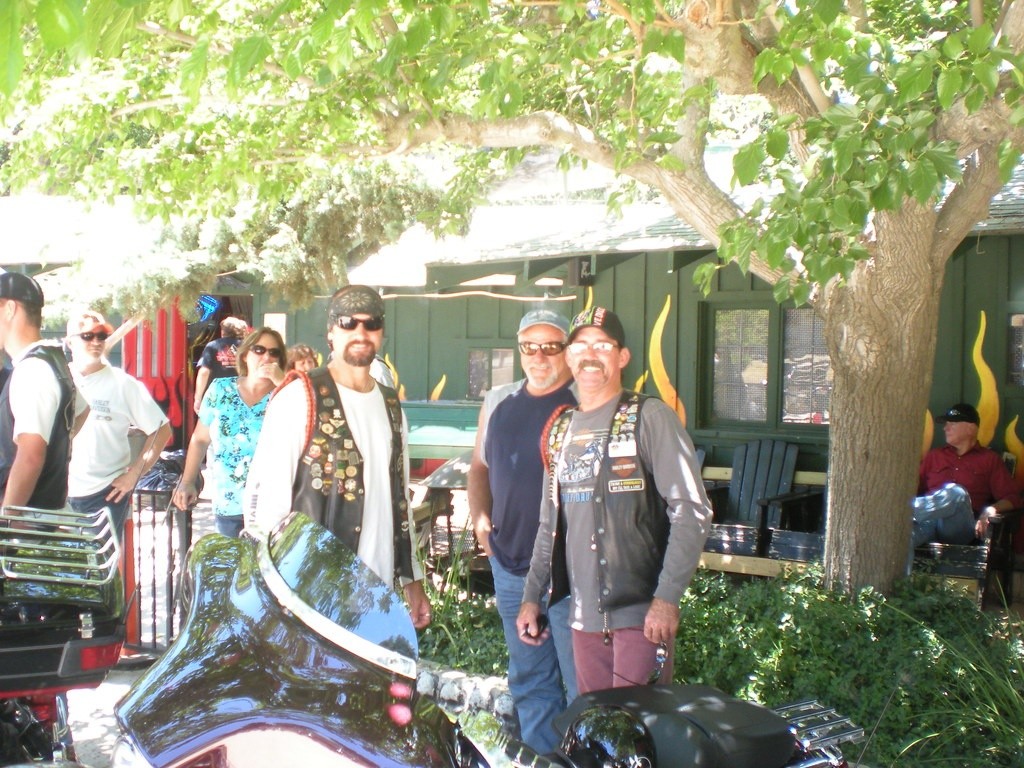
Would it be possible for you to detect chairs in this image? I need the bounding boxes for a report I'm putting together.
[914,452,1016,620]
[702,439,824,564]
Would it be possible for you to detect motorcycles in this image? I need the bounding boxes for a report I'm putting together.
[0,505,129,767]
[111,506,866,768]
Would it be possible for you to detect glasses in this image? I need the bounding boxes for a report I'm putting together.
[78,332,107,341]
[328,314,384,331]
[517,341,568,356]
[249,344,281,358]
[568,342,620,354]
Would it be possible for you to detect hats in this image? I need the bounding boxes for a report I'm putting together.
[936,404,980,424]
[569,306,625,345]
[66,310,114,340]
[516,307,571,337]
[0,272,44,308]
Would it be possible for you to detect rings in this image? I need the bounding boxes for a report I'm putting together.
[423,613,431,617]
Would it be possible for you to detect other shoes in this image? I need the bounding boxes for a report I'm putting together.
[66,744,76,762]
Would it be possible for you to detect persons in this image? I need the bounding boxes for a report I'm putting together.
[516,305,715,698]
[464,310,584,755]
[171,283,435,633]
[63,308,173,552]
[905,404,1024,575]
[0,270,90,749]
